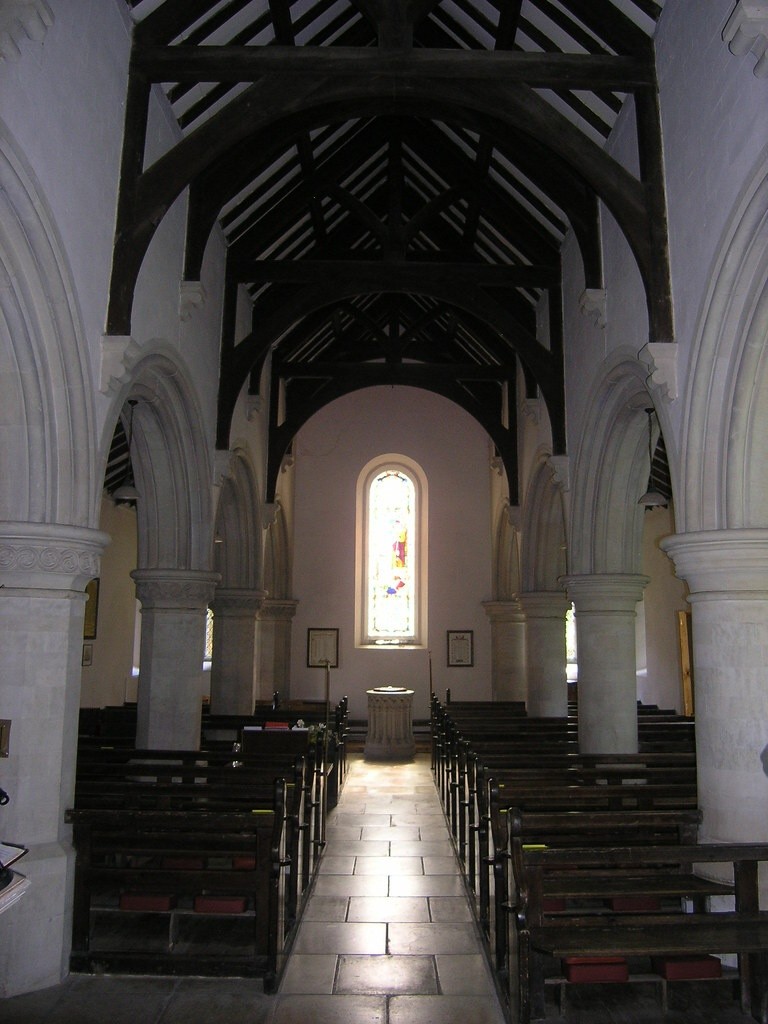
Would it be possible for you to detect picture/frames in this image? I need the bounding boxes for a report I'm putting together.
[446,630,474,667]
[84,577,100,640]
[82,644,93,667]
[307,627,339,668]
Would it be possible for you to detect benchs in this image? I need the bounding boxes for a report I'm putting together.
[429,688,768,1023]
[64,691,351,994]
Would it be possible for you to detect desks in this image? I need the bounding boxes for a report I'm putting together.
[363,687,417,758]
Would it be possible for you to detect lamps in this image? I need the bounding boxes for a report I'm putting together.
[113,398,141,501]
[636,407,667,506]
